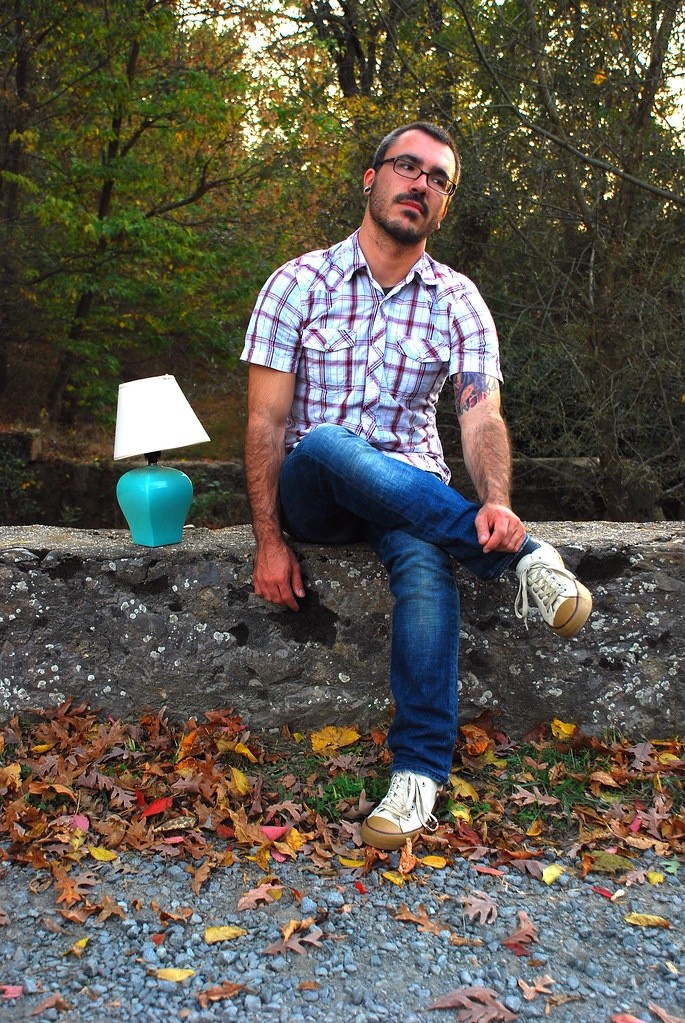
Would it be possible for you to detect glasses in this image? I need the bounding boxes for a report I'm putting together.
[373,158,457,196]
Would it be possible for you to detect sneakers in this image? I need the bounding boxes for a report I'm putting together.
[361,769,445,850]
[514,538,592,639]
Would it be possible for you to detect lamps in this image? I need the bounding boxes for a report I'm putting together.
[113,374,211,548]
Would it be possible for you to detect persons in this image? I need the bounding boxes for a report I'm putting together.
[239,122,593,851]
